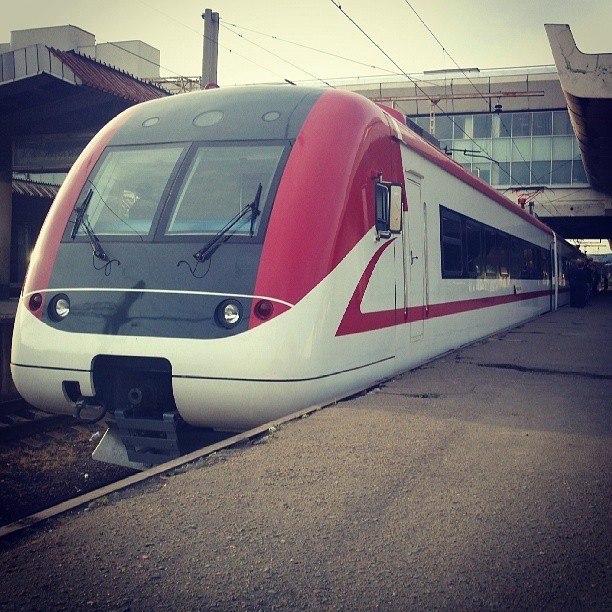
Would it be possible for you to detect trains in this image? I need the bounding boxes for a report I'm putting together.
[10,87,594,468]
